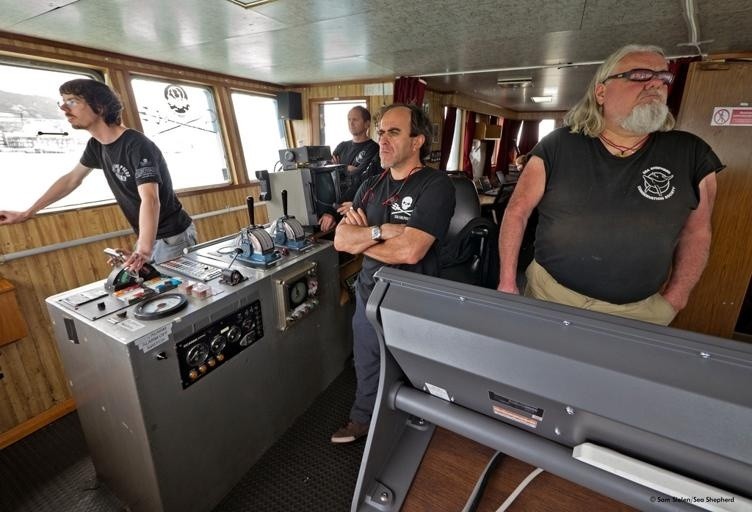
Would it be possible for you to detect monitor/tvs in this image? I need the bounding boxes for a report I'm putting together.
[348,266,751,511]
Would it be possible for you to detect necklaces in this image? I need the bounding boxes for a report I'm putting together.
[599,133,651,158]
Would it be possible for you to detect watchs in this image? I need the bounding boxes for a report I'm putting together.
[371,224,385,243]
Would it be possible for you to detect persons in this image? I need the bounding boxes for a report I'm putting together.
[0,79,197,272]
[329,103,457,445]
[318,105,384,232]
[330,106,380,195]
[496,44,726,327]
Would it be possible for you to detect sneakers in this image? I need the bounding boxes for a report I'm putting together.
[330,420,370,443]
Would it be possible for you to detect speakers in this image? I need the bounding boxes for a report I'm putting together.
[277,91,302,121]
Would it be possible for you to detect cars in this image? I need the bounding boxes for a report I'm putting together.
[1,111,76,154]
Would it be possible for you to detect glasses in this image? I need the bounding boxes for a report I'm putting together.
[602,69,676,86]
[56,100,97,108]
